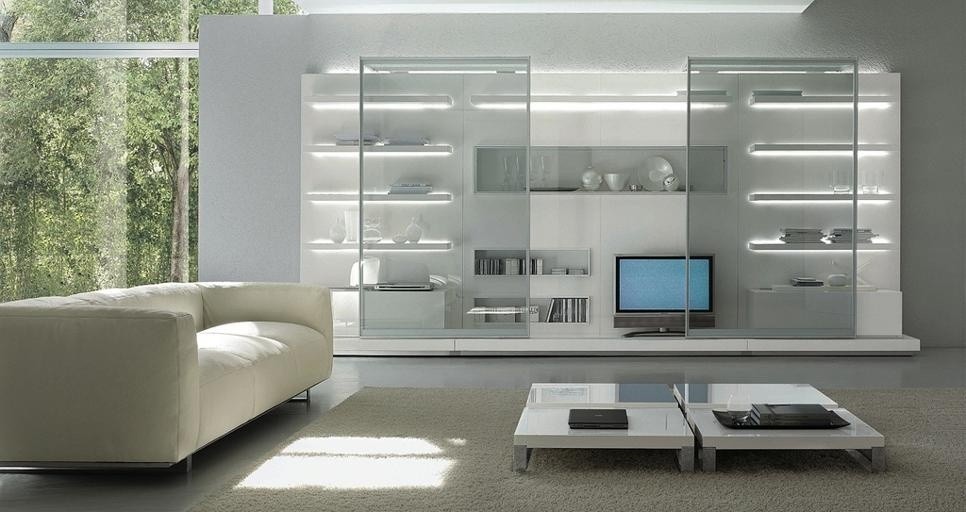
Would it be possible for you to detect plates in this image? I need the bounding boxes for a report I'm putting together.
[636,157,674,191]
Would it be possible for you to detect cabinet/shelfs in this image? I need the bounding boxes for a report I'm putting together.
[748,96,901,337]
[309,96,456,329]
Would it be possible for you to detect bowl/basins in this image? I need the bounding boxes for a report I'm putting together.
[603,173,631,191]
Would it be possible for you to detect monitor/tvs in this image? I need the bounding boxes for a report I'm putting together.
[613,253,716,337]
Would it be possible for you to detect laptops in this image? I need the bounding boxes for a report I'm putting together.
[568,409,628,429]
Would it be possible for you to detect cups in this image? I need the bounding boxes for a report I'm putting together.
[862,172,878,195]
[832,166,850,193]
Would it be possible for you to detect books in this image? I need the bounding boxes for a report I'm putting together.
[779,226,878,286]
[477,257,588,322]
[336,125,429,146]
[751,403,830,420]
[387,182,432,193]
[750,409,828,425]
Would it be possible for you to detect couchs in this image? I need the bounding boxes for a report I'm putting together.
[0,282,332,471]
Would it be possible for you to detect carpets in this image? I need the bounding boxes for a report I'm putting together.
[185,387,965,511]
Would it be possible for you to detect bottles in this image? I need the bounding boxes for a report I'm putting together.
[403,217,423,242]
[329,216,345,243]
